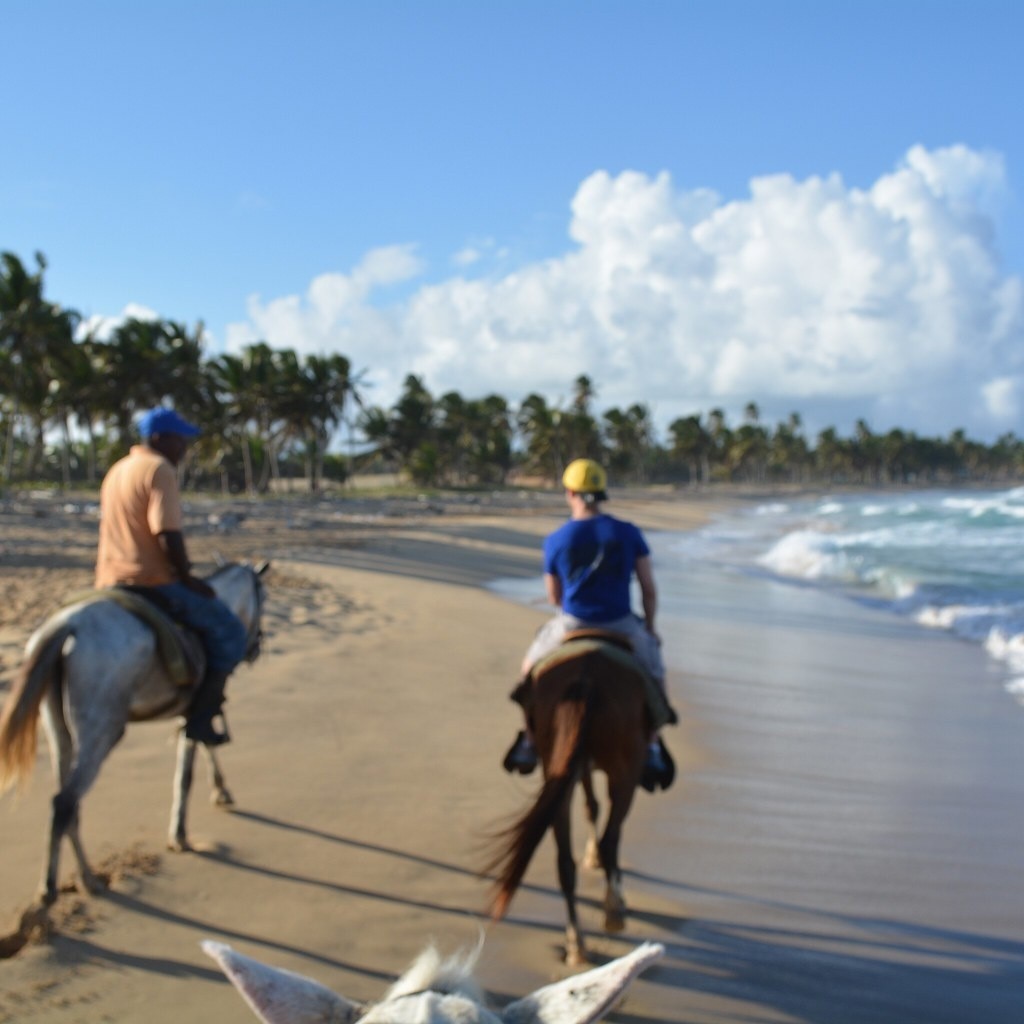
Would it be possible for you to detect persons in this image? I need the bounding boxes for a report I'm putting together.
[97,409,247,745]
[503,460,676,791]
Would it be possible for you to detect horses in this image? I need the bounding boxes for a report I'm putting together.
[481,614,667,966]
[201,938,666,1024]
[0,553,270,903]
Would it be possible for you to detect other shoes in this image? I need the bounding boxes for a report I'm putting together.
[515,743,535,774]
[647,740,665,775]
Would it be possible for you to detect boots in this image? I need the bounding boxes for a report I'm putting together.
[187,655,230,747]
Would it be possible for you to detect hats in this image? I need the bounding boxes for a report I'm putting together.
[562,459,608,506]
[137,408,201,440]
